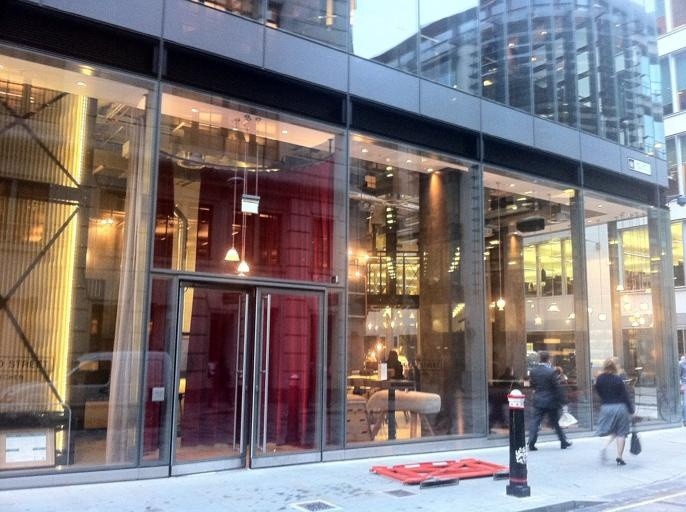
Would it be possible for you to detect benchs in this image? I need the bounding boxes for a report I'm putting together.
[367,390,442,440]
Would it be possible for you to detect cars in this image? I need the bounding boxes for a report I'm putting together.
[0,350,172,427]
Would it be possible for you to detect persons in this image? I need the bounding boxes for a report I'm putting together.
[528,352,573,450]
[679,356,686,426]
[596,358,635,465]
[498,366,516,386]
[381,351,404,380]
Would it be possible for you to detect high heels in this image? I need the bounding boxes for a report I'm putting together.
[614,457,626,467]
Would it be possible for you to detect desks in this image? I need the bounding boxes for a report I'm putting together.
[348,375,415,425]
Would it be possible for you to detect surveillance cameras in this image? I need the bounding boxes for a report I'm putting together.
[677,196,686,206]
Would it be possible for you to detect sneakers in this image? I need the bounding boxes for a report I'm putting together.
[560,441,572,450]
[525,441,538,451]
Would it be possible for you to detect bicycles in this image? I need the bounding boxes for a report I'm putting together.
[658,387,685,424]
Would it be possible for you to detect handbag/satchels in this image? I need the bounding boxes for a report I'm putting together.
[629,418,641,456]
